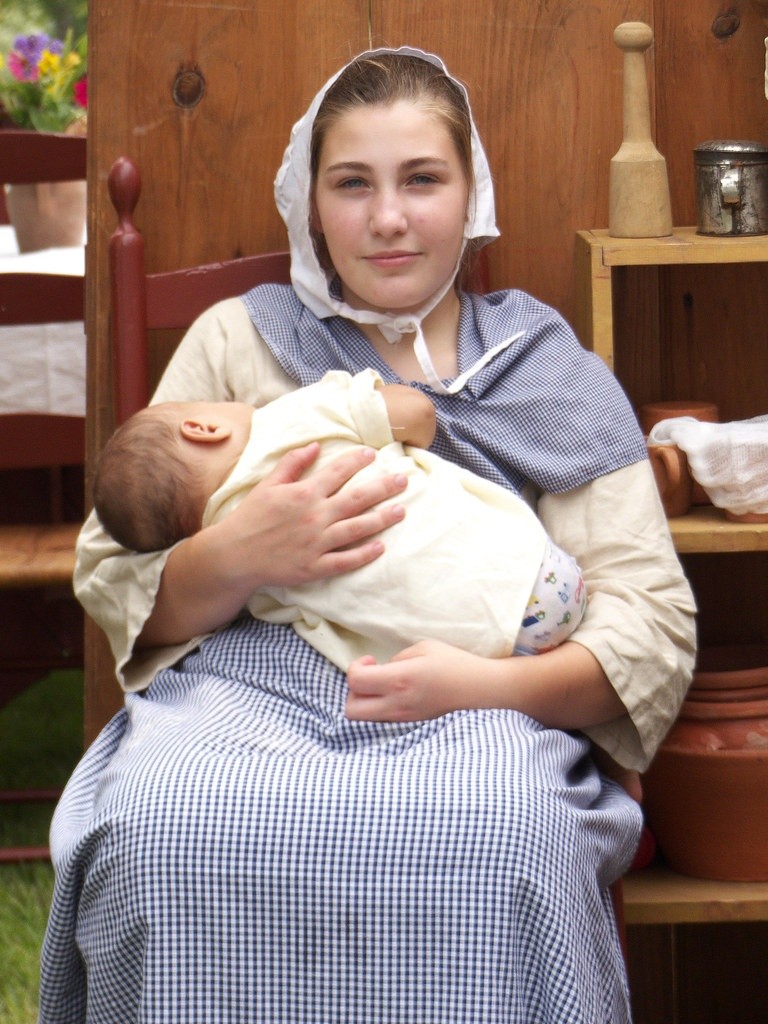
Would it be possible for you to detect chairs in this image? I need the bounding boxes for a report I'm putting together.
[0,133,88,861]
[108,156,489,428]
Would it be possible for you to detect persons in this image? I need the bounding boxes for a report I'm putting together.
[37,44,700,1024]
[93,365,645,803]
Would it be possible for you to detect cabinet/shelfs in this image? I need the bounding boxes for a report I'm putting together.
[575,226,768,924]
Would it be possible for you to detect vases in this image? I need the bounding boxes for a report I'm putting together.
[9,183,84,249]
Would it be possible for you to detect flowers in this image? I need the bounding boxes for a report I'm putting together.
[1,25,88,134]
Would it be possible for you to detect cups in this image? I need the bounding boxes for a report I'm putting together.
[640,434,693,518]
[638,402,722,506]
[692,139,768,237]
